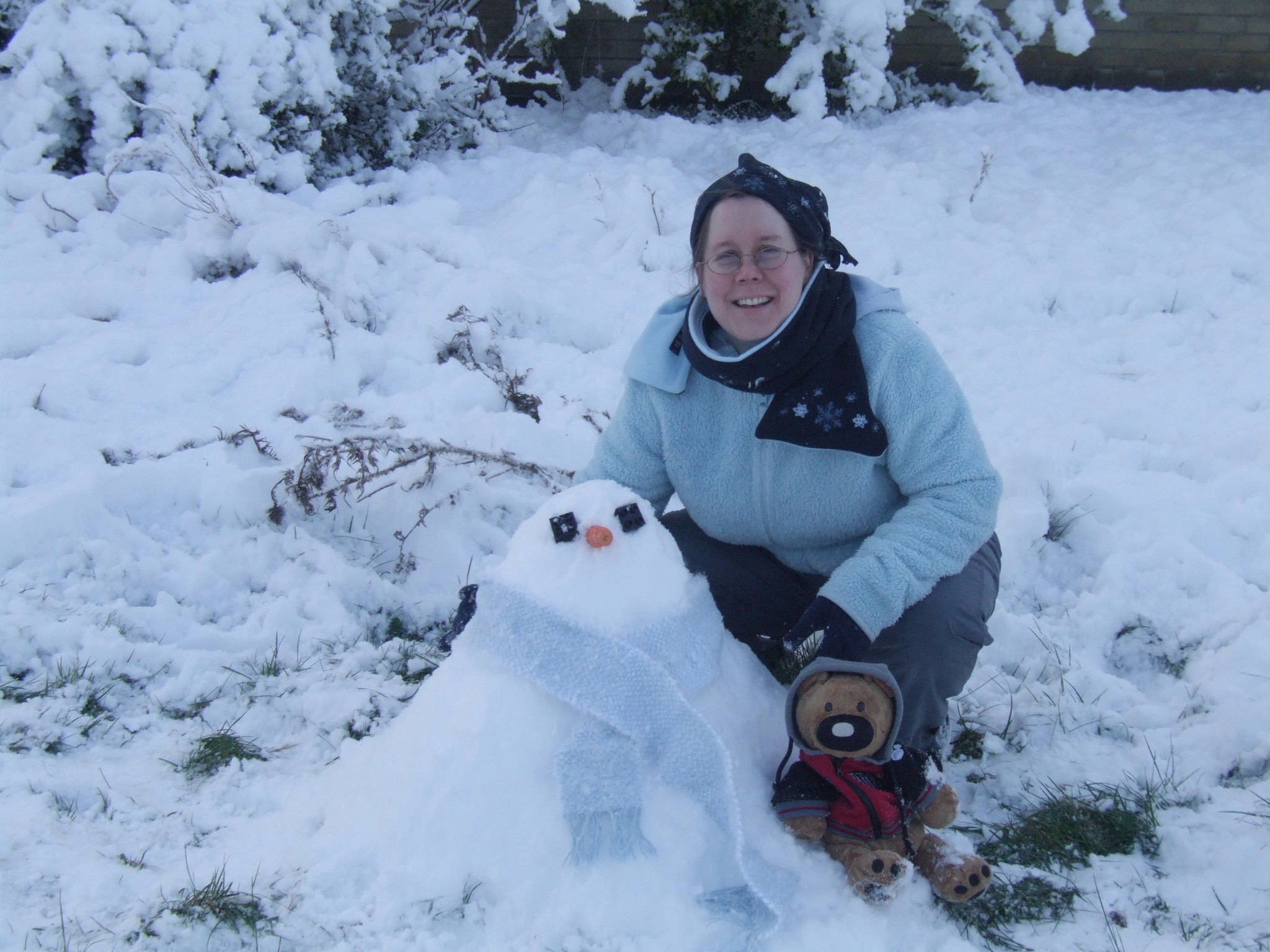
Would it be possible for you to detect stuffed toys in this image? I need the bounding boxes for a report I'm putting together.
[771,658,991,906]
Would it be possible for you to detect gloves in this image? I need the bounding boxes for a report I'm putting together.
[783,592,871,668]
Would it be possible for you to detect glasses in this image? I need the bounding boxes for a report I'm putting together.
[699,245,801,275]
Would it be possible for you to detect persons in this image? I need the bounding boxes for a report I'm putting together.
[568,155,1003,776]
